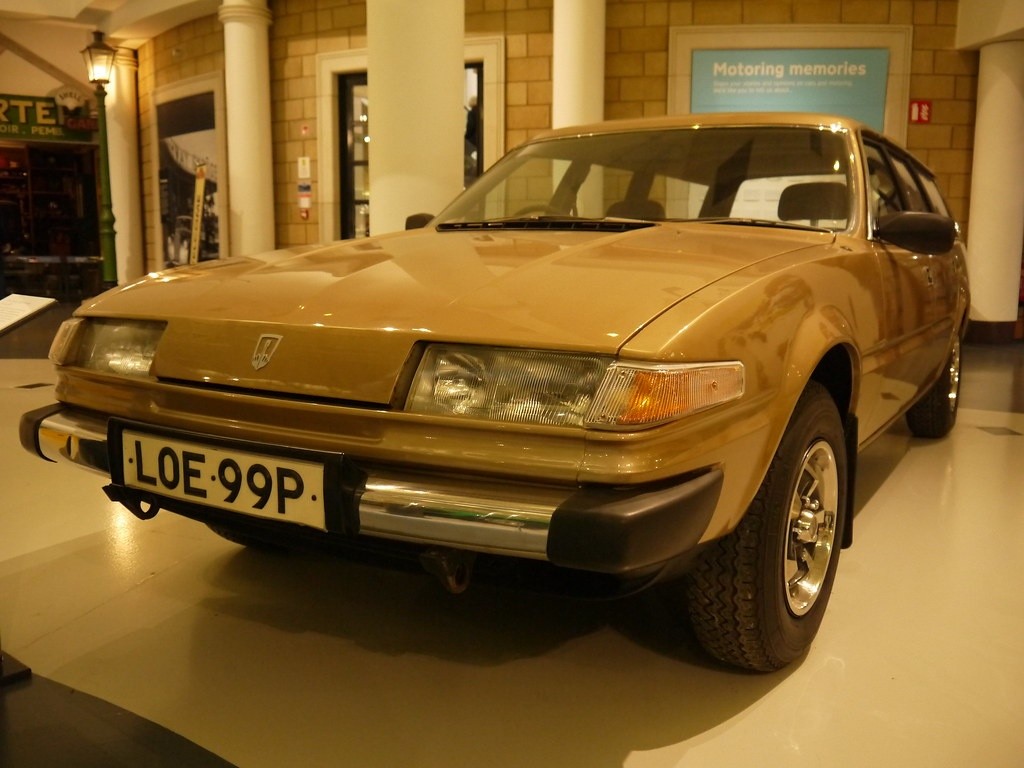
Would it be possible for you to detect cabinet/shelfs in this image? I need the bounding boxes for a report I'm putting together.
[0,139,101,300]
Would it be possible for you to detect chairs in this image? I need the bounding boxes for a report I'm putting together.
[777,183,849,226]
[606,200,664,220]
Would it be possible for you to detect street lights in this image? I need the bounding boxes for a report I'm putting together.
[79,29,117,287]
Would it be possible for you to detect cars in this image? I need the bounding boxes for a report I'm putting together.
[18,110,971,673]
